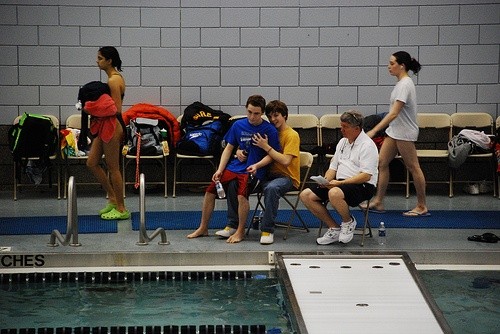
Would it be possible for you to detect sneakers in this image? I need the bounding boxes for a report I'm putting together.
[216,226,237,238]
[339,214,357,243]
[260,232,274,244]
[316,228,341,245]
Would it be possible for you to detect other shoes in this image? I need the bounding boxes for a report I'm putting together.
[462,183,479,194]
[479,182,494,193]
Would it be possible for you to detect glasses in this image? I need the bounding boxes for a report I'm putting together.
[344,112,359,121]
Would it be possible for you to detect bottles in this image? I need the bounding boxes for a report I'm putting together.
[215,177,225,199]
[378,222,387,246]
[259,208,264,230]
[161,128,167,138]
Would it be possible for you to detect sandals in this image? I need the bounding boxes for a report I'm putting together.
[99,203,117,216]
[101,208,130,220]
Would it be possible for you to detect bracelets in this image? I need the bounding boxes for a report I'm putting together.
[267,148,272,152]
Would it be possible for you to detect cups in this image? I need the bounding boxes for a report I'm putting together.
[253,216,258,229]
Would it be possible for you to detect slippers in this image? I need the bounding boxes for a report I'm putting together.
[360,204,385,213]
[468,233,498,243]
[402,210,431,217]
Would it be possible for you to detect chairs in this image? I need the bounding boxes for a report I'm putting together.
[244,151,314,241]
[11,114,61,202]
[319,113,342,177]
[171,114,218,198]
[286,113,320,184]
[317,157,375,248]
[450,112,497,198]
[406,112,452,198]
[63,114,110,199]
[496,115,500,200]
[121,102,168,198]
[363,112,408,198]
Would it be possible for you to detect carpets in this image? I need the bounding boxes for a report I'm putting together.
[258,208,500,230]
[129,210,262,230]
[0,215,118,236]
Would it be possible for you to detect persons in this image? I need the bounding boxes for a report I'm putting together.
[360,51,431,217]
[186,95,281,244]
[87,46,130,219]
[236,99,301,243]
[300,111,379,245]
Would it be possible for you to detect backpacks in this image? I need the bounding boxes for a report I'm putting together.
[130,117,163,155]
[8,112,58,159]
[181,121,220,156]
